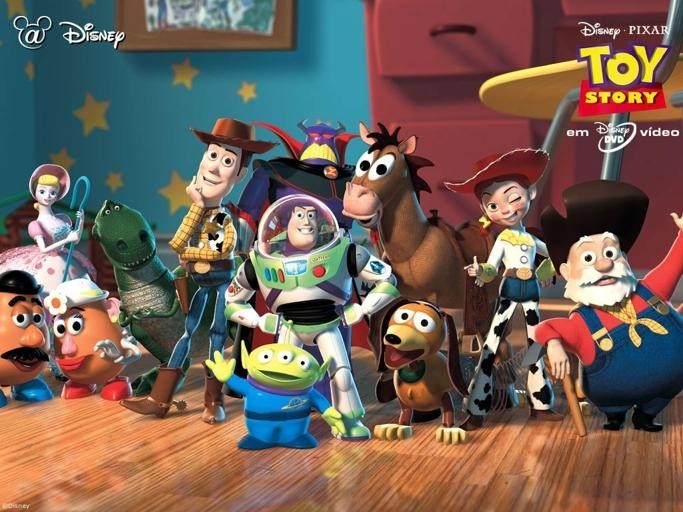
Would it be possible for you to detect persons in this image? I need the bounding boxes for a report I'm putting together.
[0,114,683,450]
